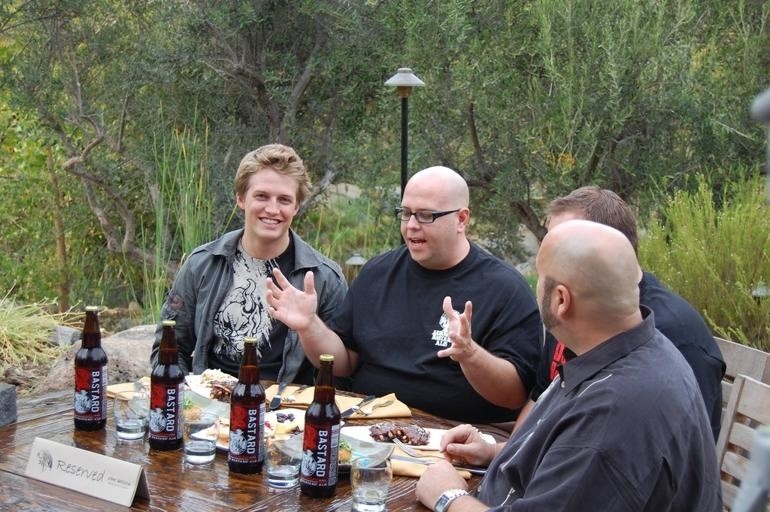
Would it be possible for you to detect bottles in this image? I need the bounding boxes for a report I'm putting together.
[73,304,109,431]
[149,320,184,452]
[300,353,341,497]
[228,337,266,475]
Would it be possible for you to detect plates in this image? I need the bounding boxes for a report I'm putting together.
[274,431,396,468]
[189,407,345,452]
[340,425,496,451]
[185,372,240,404]
[128,389,231,425]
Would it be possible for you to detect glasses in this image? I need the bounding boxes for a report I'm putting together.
[395,207,460,223]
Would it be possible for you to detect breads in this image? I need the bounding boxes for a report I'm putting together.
[184,402,201,420]
[338,442,351,463]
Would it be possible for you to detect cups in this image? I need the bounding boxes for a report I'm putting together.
[349,455,393,512]
[113,390,150,439]
[265,435,303,489]
[180,412,219,465]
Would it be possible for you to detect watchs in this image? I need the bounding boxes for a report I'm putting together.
[433,489,468,511]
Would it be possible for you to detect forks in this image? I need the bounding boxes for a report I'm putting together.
[393,438,444,457]
[284,384,308,402]
[351,399,393,416]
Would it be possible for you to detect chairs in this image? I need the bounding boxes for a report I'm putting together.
[716,372,769,512]
[710,334,769,491]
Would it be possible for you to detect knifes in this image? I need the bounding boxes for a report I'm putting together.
[269,381,287,410]
[393,454,486,477]
[341,395,376,419]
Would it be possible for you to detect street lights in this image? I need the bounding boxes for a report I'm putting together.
[382,68,426,245]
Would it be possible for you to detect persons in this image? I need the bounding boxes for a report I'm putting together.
[508,187,726,446]
[416,219,723,510]
[265,166,544,424]
[149,144,349,387]
